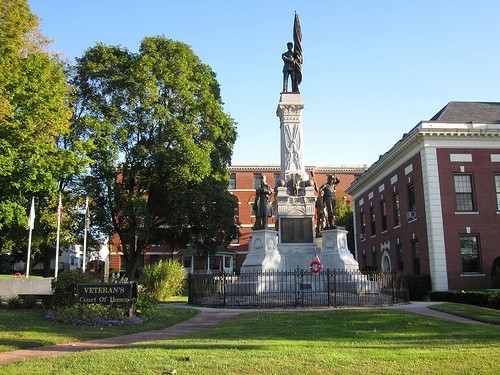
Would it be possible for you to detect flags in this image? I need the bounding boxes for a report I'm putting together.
[57,195,63,224]
[85,200,90,228]
[27,200,36,229]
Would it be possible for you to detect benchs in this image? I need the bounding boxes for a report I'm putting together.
[17,293,55,307]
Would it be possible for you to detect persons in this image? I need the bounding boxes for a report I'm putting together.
[280,41,301,93]
[255,175,273,230]
[317,173,342,229]
[314,187,327,237]
